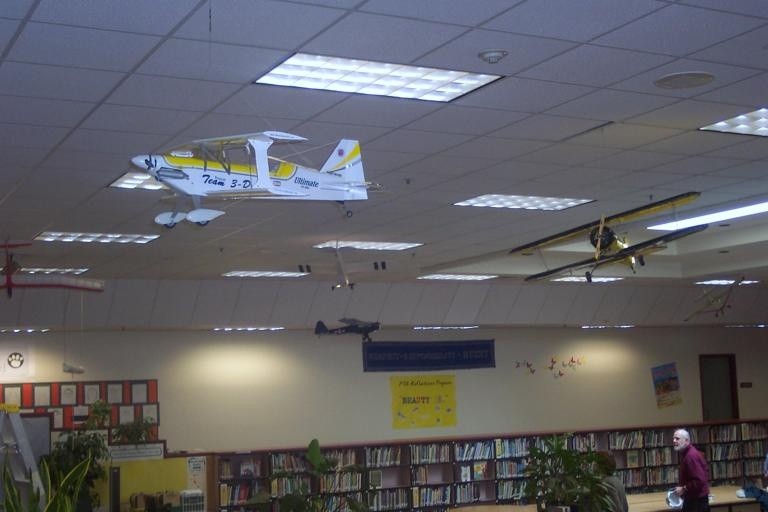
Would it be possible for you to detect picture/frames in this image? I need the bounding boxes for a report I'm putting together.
[3,380,159,449]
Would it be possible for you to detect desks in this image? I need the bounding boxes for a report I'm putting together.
[444,485,761,512]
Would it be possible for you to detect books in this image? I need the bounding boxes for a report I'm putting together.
[608,431,679,487]
[271,448,312,496]
[366,446,402,468]
[368,470,382,489]
[217,457,262,508]
[572,433,596,476]
[366,488,409,512]
[689,423,768,486]
[409,436,567,505]
[319,447,363,512]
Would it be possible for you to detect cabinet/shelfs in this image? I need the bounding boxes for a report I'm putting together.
[496,433,571,504]
[206,449,271,512]
[364,441,412,512]
[411,438,455,510]
[269,442,367,512]
[644,418,767,494]
[572,430,648,495]
[453,436,497,506]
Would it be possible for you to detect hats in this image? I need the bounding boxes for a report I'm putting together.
[666,488,684,509]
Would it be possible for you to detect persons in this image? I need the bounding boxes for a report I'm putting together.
[672,429,711,512]
[585,451,628,512]
